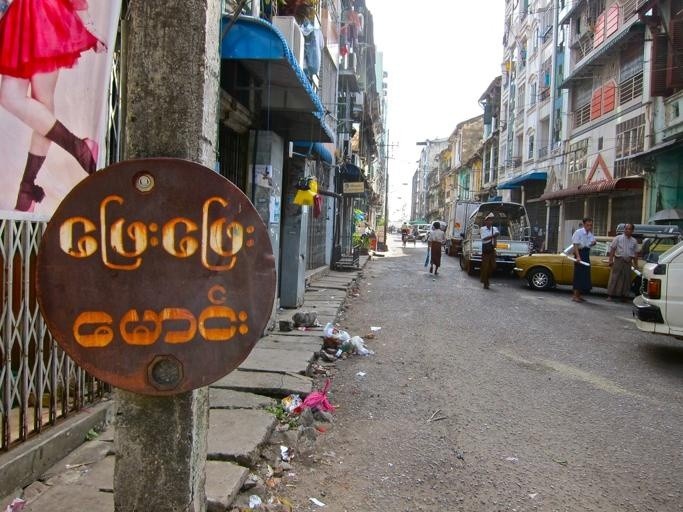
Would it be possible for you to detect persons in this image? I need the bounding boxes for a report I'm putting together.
[0,0,100,220]
[572,218,595,302]
[400,222,410,243]
[606,223,641,300]
[480,216,500,290]
[428,223,447,274]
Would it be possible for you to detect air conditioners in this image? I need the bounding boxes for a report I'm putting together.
[271,12,306,73]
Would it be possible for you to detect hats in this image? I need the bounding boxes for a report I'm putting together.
[483,212,495,220]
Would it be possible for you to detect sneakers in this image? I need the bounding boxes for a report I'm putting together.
[434,268,439,275]
[429,266,433,273]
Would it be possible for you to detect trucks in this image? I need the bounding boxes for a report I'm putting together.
[410,198,680,298]
[629,236,682,338]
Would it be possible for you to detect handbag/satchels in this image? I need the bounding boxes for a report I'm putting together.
[423,253,430,267]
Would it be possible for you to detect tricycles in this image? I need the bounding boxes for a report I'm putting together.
[401,228,417,248]
